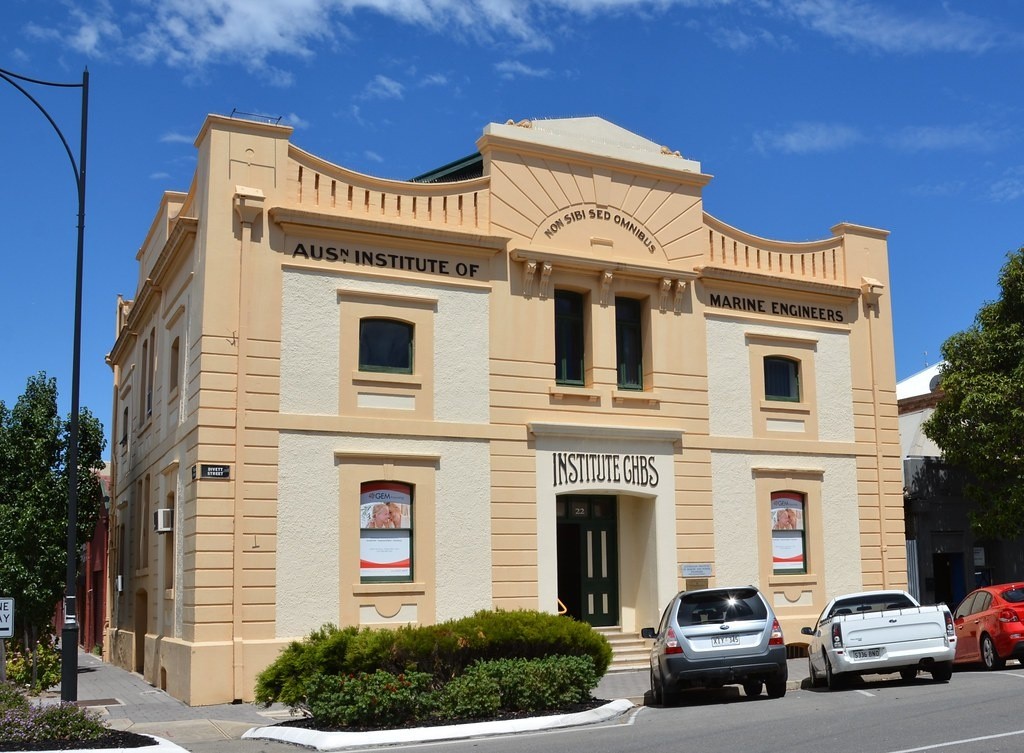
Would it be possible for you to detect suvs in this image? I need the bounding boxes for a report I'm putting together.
[641,584,788,708]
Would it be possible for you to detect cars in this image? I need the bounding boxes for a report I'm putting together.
[951,580,1024,672]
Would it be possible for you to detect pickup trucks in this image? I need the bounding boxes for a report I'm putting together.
[801,589,957,690]
